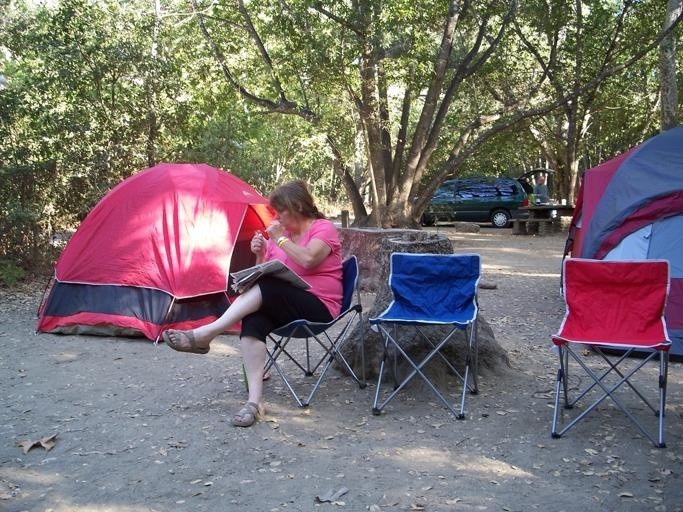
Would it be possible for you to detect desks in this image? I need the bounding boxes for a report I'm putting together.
[516,203,576,234]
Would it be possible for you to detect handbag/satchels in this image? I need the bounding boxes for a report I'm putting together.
[523,193,536,208]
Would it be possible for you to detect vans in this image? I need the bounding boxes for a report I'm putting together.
[411,168,555,227]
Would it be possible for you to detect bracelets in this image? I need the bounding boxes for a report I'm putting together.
[277,236,289,248]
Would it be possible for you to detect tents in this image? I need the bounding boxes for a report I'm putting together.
[560,126,683,355]
[35,163,276,344]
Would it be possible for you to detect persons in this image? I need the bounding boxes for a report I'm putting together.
[534,175,549,203]
[162,179,344,426]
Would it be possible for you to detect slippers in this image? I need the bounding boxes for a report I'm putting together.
[232,401,269,427]
[162,329,210,354]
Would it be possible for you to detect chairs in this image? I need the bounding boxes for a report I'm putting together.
[548,252,674,449]
[225,252,367,411]
[362,250,486,421]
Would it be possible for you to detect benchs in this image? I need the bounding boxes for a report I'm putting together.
[508,216,555,236]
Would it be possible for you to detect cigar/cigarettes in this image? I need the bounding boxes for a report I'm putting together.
[265,225,272,232]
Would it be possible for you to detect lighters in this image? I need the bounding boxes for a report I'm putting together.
[259,233,263,237]
